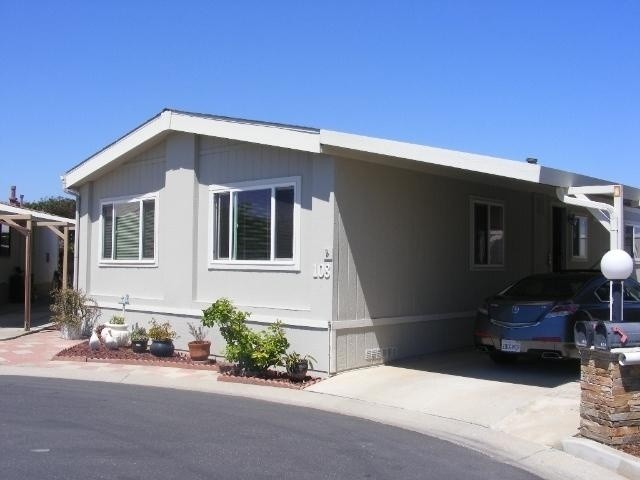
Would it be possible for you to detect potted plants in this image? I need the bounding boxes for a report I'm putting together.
[46,282,319,381]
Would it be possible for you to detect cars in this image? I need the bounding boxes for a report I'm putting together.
[474,268,640,363]
